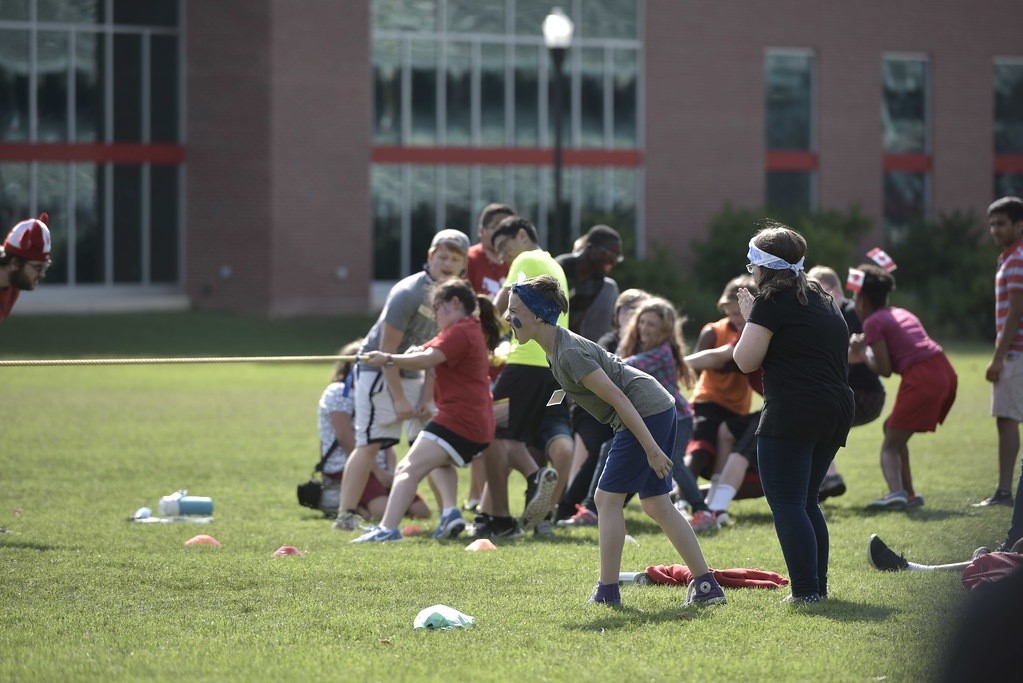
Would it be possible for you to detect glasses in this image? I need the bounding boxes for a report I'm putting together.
[27,262,50,272]
[596,246,624,263]
[746,263,755,273]
[496,235,513,258]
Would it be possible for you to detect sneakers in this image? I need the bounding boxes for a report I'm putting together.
[334,513,377,531]
[434,509,465,539]
[587,581,620,606]
[556,500,599,525]
[818,474,846,503]
[533,520,560,544]
[864,491,925,513]
[521,466,560,531]
[466,513,525,539]
[867,534,909,572]
[462,503,481,515]
[674,501,730,536]
[684,572,727,606]
[349,527,402,542]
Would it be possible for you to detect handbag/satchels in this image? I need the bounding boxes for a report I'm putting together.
[298,481,322,508]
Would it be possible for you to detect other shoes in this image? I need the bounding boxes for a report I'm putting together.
[780,595,829,605]
[972,492,1014,508]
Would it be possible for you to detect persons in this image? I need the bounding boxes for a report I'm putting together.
[0,212,53,323]
[866,458,1023,683]
[505,272,730,609]
[733,226,854,606]
[319,203,959,544]
[971,197,1023,506]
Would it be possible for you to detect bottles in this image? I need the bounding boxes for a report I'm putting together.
[161,492,213,515]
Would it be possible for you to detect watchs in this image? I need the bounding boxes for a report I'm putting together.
[383,352,395,367]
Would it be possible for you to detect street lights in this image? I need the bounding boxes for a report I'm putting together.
[541,6,577,257]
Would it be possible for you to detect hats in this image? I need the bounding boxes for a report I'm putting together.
[431,229,470,278]
[585,226,624,253]
[5,213,51,261]
